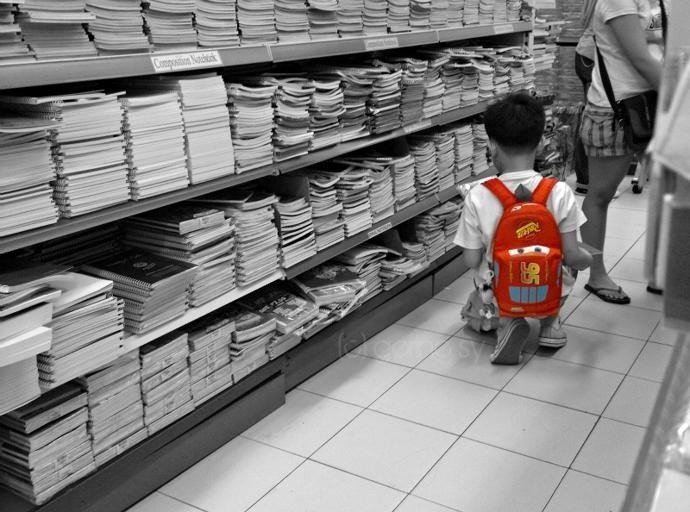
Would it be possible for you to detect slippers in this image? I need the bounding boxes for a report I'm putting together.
[585,276,632,305]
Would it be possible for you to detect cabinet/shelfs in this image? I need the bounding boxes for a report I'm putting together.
[0,0,559,512]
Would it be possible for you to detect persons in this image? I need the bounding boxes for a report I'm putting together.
[584,0,665,304]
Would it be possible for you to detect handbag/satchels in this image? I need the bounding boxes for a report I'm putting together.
[614,90,659,152]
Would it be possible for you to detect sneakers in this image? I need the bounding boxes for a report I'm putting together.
[538,318,568,348]
[488,314,530,366]
[573,183,620,199]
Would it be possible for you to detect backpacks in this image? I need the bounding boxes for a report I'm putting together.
[481,175,562,319]
